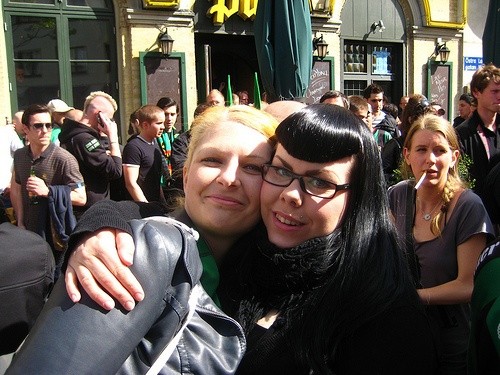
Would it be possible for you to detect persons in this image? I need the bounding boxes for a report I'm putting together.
[0,64,500,375]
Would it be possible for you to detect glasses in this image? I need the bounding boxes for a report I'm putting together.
[33,123,54,130]
[260,162,352,199]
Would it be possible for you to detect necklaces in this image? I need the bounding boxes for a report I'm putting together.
[420,195,443,221]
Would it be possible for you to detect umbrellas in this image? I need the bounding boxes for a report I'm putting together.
[253,0,315,101]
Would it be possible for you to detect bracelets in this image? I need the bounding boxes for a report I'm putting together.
[109,141,116,144]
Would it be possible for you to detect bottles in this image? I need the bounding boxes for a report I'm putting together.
[27,170,40,206]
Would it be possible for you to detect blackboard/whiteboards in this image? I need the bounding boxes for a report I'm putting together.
[427,60,451,123]
[307,55,335,104]
[139,51,188,133]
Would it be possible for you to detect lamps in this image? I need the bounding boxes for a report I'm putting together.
[372,20,386,33]
[158,27,174,58]
[313,34,329,62]
[435,41,450,65]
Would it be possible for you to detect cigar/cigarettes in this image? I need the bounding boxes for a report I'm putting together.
[414,172,427,190]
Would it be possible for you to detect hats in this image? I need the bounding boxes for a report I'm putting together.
[47,99,75,113]
